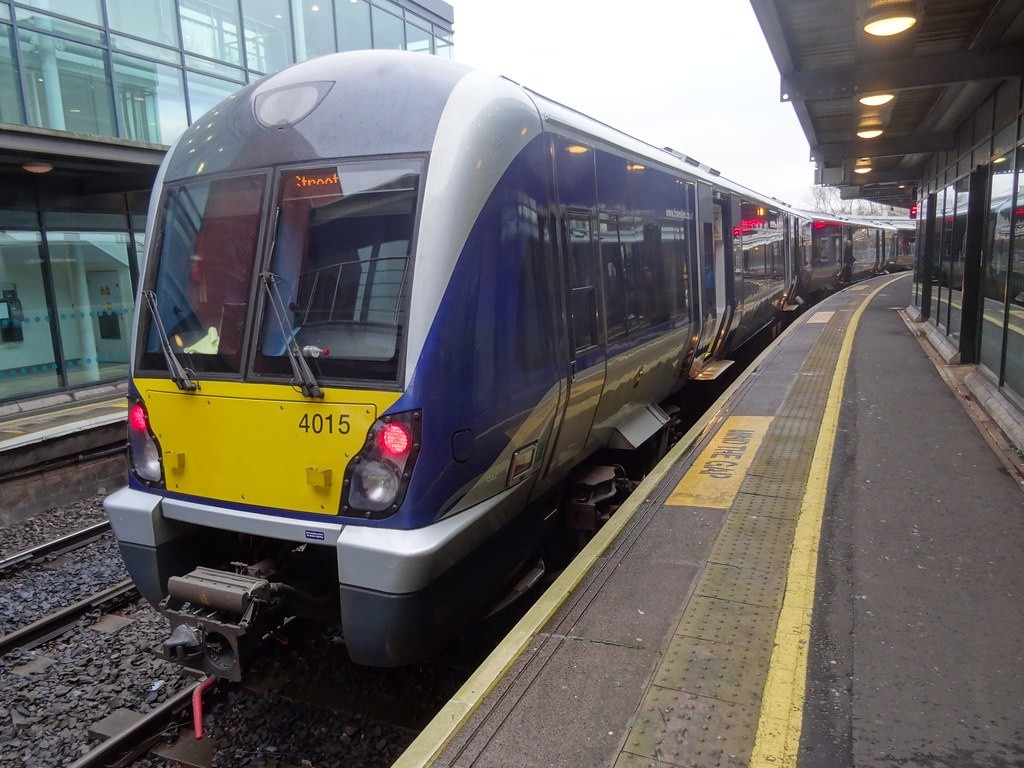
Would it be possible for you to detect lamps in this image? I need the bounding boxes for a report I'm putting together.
[852,0,927,44]
[24,162,55,175]
[853,156,874,176]
[854,91,898,140]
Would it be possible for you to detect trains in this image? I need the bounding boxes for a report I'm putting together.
[101,48,916,687]
